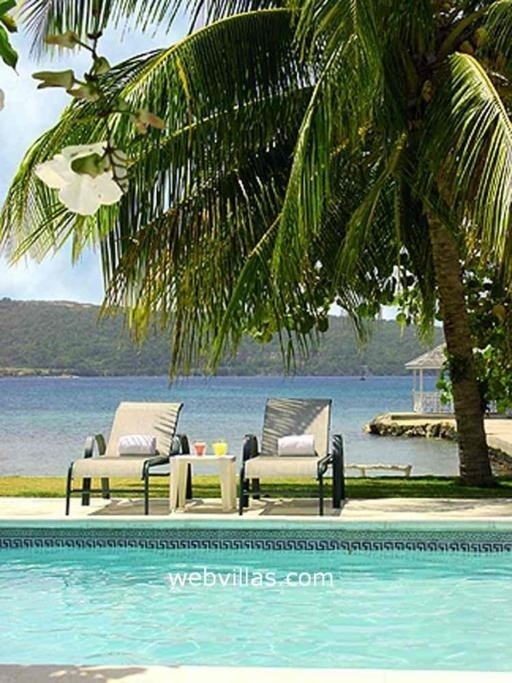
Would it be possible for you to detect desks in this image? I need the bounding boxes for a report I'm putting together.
[173,453,240,512]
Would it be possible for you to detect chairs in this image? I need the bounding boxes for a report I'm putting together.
[236,396,347,517]
[63,399,192,515]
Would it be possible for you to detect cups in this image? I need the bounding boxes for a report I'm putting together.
[193,440,228,456]
